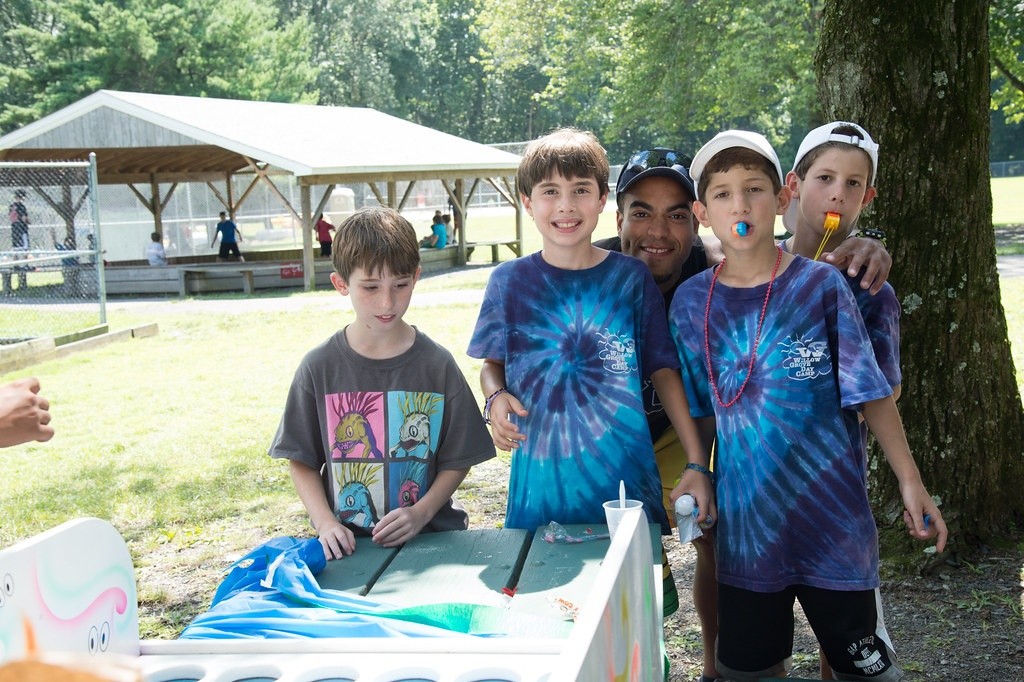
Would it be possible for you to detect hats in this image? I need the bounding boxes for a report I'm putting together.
[689,131,784,201]
[618,147,695,199]
[783,122,879,234]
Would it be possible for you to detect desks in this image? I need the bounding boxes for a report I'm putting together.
[174,521,609,641]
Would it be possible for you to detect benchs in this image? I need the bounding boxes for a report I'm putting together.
[0,238,518,294]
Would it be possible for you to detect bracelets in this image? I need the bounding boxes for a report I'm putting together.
[483,387,510,426]
[685,463,716,482]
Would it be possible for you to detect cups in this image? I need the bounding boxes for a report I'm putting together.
[602,500,643,546]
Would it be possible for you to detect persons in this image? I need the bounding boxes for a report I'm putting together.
[313,213,336,260]
[53,236,80,266]
[466,127,717,682]
[8,189,36,271]
[418,178,468,251]
[146,232,169,266]
[591,120,949,682]
[87,234,108,267]
[0,376,55,448]
[210,211,246,263]
[267,205,497,563]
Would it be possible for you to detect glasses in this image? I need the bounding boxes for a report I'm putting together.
[616,151,692,190]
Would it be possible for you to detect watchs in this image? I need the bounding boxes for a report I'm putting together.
[847,228,893,256]
